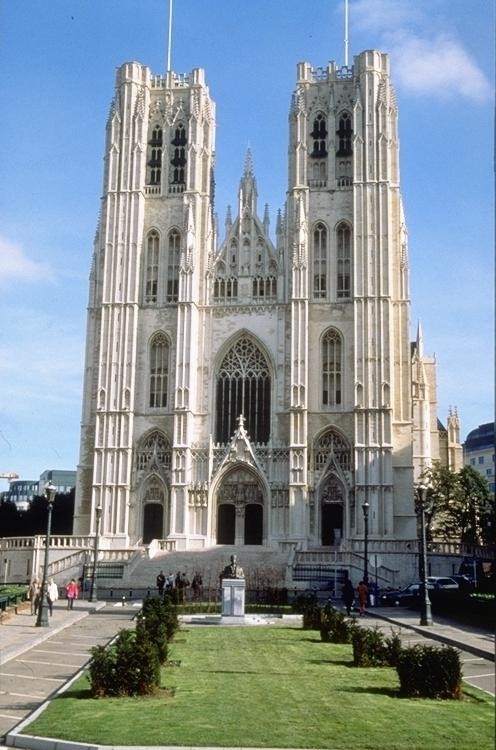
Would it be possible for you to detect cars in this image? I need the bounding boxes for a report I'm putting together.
[380,572,478,609]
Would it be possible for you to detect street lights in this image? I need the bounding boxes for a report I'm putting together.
[360,501,373,608]
[416,481,436,628]
[86,501,106,603]
[34,481,59,627]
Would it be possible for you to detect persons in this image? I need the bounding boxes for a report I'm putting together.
[174,570,187,600]
[156,570,166,595]
[181,572,191,598]
[356,581,369,616]
[46,578,58,616]
[164,572,173,593]
[341,579,355,616]
[191,570,204,602]
[64,578,78,610]
[26,579,40,615]
[222,554,245,579]
